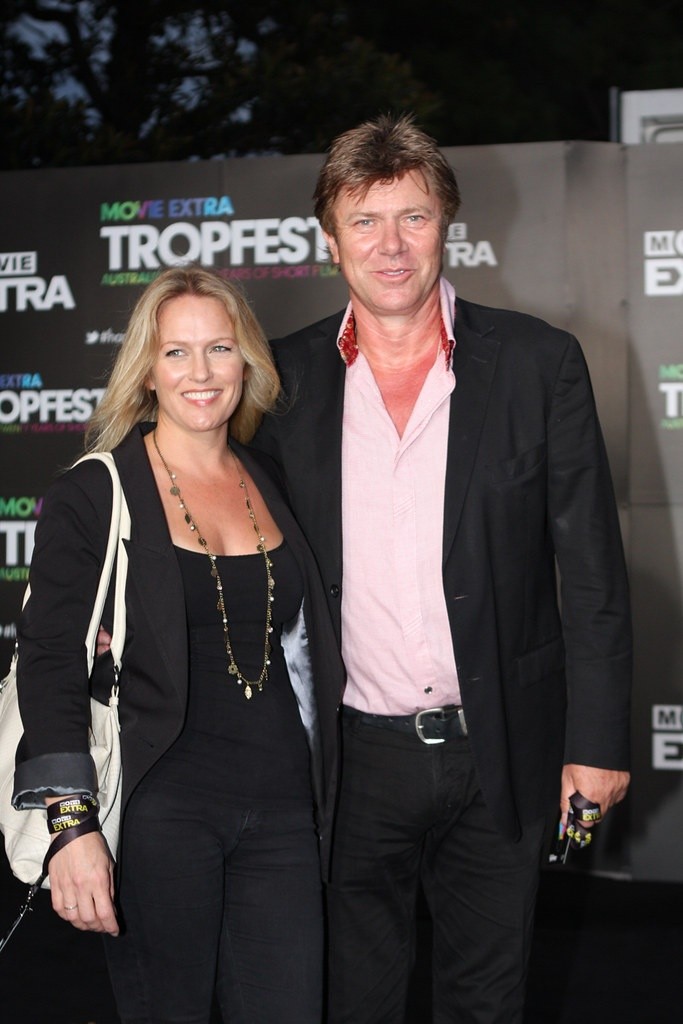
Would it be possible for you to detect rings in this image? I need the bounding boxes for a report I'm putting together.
[65,905,78,910]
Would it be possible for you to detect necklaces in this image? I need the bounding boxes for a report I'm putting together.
[153,429,275,700]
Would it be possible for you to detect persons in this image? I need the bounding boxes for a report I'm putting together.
[97,120,635,1024]
[11,270,347,1024]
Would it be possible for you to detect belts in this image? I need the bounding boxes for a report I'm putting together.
[341,708,467,741]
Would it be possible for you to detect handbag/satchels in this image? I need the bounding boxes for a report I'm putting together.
[0,454,128,888]
